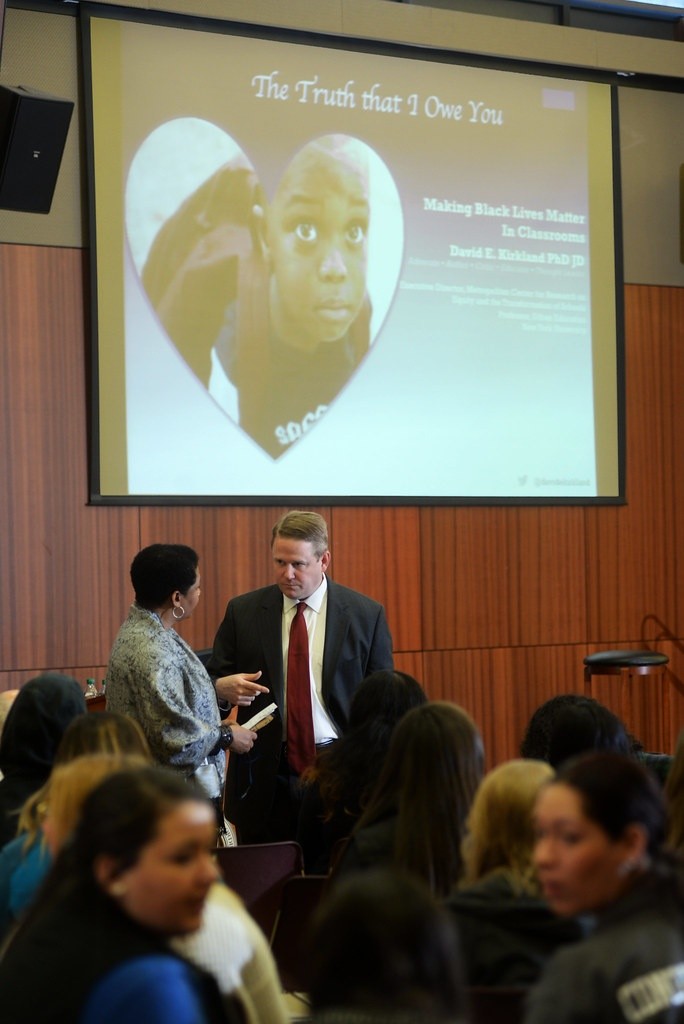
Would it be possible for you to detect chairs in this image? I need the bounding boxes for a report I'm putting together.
[210,841,351,990]
[441,896,582,1024]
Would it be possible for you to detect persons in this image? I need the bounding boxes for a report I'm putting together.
[0,671,684,1023]
[205,510,393,842]
[140,142,383,461]
[106,544,258,833]
[0,764,235,1024]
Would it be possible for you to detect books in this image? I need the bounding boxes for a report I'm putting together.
[240,701,276,733]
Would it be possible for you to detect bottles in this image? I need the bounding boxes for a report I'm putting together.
[83,678,98,698]
[99,680,106,695]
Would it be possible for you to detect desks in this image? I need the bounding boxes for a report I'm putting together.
[85,694,106,712]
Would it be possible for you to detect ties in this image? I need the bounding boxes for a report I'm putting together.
[287,603,315,775]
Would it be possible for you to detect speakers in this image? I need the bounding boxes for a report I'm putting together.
[0,84,74,215]
